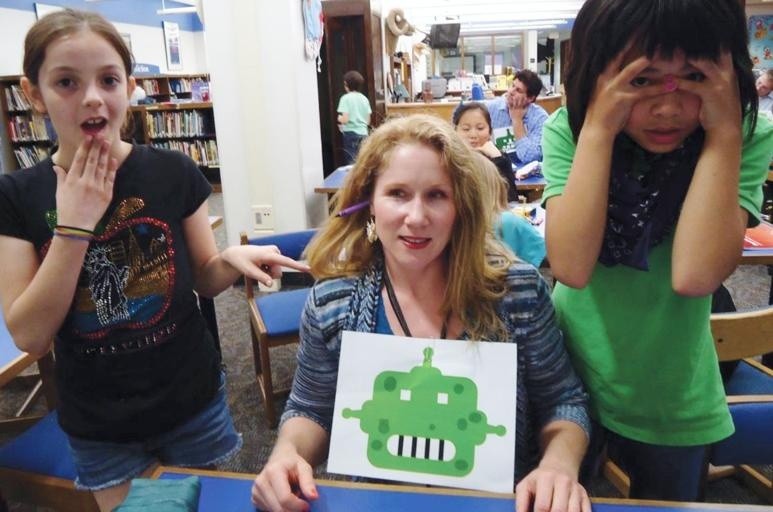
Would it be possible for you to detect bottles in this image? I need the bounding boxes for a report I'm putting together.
[462,83,484,100]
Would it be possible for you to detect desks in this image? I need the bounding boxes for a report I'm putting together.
[739,214,773,306]
[111,466,773,512]
[314,166,547,204]
[0,215,221,436]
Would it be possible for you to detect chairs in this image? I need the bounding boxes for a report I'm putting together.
[710,396,773,467]
[603,307,773,497]
[240,231,313,429]
[0,407,97,512]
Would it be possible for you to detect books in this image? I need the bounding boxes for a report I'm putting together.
[4,85,50,169]
[142,77,220,169]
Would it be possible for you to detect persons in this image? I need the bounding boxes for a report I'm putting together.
[452,101,519,203]
[0,10,311,512]
[251,114,593,512]
[540,0,773,502]
[746,69,773,120]
[337,70,373,164]
[487,69,549,164]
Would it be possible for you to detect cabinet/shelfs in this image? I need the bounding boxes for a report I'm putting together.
[0,72,222,194]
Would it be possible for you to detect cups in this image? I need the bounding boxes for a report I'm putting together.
[390,97,397,103]
[420,80,432,103]
[403,97,412,103]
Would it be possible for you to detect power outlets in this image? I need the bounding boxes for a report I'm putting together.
[252,205,274,230]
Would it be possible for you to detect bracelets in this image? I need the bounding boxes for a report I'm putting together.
[54,225,94,241]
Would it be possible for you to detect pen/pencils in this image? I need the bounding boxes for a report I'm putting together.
[335,199,369,217]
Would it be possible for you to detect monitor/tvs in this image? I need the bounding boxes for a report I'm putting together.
[429,22,461,49]
